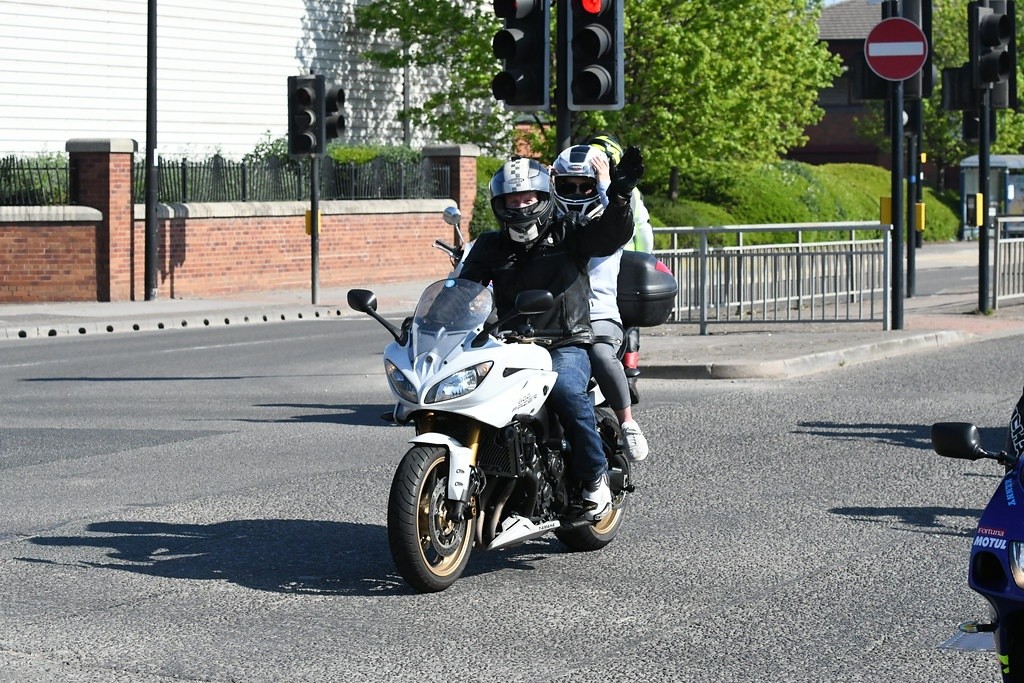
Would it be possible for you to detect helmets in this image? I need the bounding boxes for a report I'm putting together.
[487,157,554,244]
[551,145,609,221]
[581,133,623,168]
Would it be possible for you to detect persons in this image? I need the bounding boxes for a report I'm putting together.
[402,144,645,518]
[547,144,648,464]
[588,135,653,405]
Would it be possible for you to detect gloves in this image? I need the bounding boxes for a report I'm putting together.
[606,143,643,206]
[400,315,427,334]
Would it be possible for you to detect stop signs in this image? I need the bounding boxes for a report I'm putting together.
[862,16,928,83]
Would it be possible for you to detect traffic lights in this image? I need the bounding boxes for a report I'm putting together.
[567,0,626,112]
[288,75,326,158]
[971,5,1012,91]
[492,0,550,112]
[323,82,348,145]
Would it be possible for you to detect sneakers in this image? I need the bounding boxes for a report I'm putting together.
[620,420,649,461]
[582,470,612,520]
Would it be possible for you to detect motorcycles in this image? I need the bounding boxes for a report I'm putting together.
[346,250,680,595]
